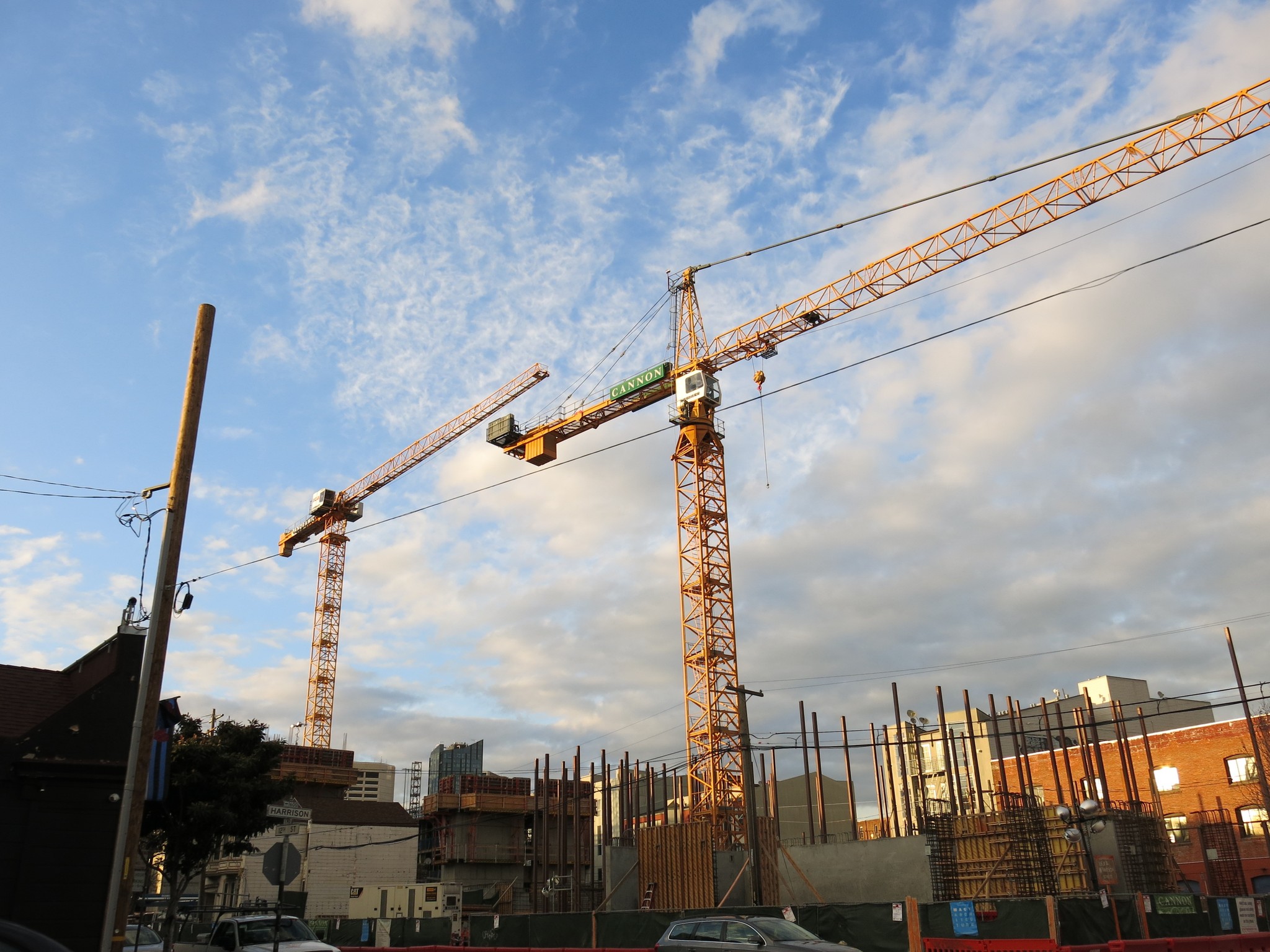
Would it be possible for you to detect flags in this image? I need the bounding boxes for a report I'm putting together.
[146,699,178,801]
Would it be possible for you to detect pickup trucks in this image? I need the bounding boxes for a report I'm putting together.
[173,903,340,952]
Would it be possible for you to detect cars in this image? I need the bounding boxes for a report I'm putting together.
[122,924,163,952]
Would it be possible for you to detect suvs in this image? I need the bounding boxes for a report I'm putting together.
[655,914,862,952]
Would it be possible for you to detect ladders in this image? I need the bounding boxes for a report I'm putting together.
[640,882,657,912]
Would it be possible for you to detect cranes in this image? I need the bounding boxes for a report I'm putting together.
[485,77,1270,908]
[275,364,548,748]
